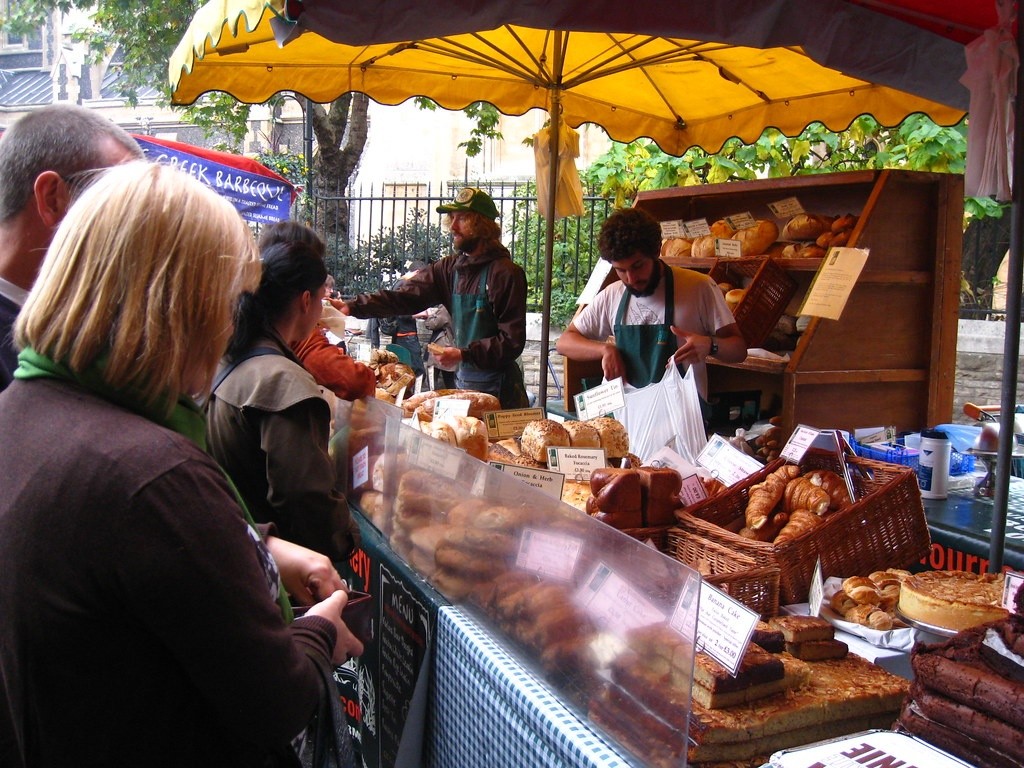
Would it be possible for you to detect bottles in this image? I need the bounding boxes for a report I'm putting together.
[919,430,951,499]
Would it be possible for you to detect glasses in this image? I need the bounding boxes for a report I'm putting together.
[233,288,256,332]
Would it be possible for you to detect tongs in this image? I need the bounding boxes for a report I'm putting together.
[830,429,873,506]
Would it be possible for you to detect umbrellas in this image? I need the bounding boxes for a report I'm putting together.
[169,0,969,420]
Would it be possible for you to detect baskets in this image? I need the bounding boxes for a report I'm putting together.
[673,446,933,608]
[707,254,798,350]
[594,525,781,624]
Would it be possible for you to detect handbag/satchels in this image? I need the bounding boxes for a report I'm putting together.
[602,351,708,466]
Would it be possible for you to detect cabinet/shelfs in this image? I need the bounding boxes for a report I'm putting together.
[564,169,965,452]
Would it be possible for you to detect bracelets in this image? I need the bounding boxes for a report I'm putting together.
[709,336,717,354]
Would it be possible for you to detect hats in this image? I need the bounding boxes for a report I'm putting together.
[437,187,500,220]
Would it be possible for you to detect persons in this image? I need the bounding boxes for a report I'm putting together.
[557,209,748,418]
[380,260,456,392]
[0,103,145,391]
[327,188,527,407]
[1,160,363,768]
[205,221,377,562]
[321,274,346,354]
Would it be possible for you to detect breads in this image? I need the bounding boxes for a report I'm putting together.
[329,342,1024,768]
[661,211,857,349]
